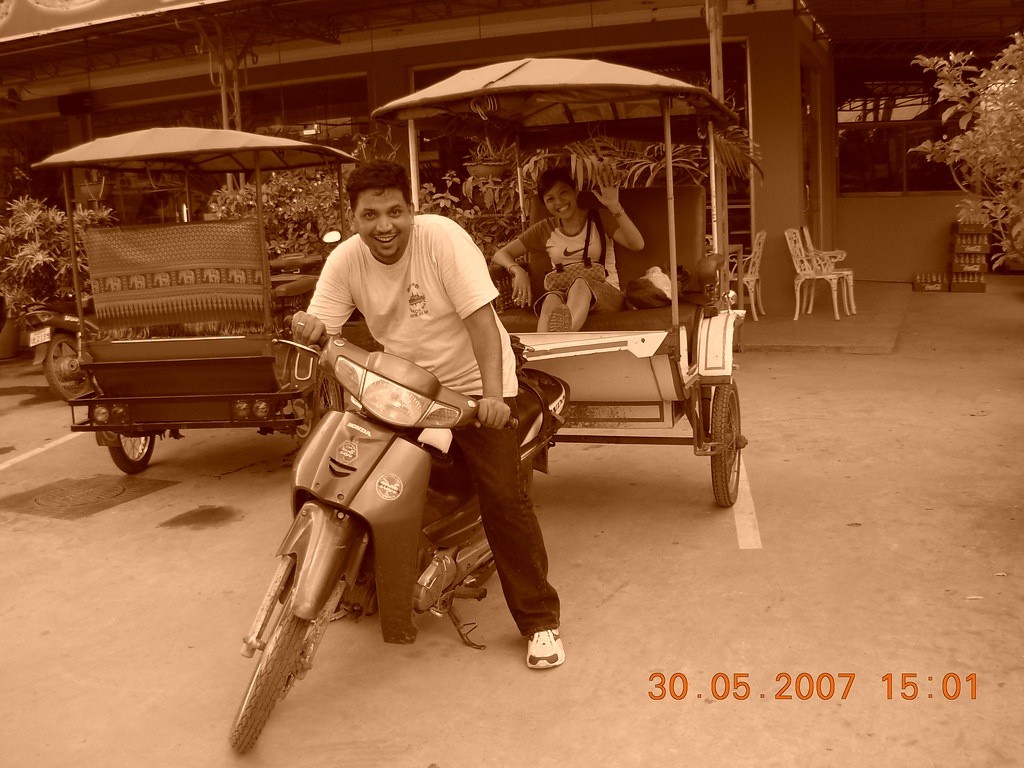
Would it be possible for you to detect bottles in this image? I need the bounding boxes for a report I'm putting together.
[912,271,948,284]
[951,205,990,286]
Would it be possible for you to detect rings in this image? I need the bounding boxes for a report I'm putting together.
[297,322,305,326]
[518,291,522,295]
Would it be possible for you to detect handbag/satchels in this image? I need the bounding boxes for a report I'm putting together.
[544,257,606,291]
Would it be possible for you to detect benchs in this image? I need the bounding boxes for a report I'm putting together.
[489,184,726,355]
[81,217,314,340]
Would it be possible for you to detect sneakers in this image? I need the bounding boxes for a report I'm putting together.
[526,628,565,669]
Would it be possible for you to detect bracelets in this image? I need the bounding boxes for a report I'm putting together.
[613,208,626,219]
[508,263,519,276]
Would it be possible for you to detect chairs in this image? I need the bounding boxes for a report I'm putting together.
[784,228,850,321]
[800,225,857,315]
[729,230,766,321]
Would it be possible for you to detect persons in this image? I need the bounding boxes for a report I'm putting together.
[493,167,645,332]
[291,159,565,669]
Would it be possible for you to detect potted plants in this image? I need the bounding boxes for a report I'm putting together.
[462,136,516,181]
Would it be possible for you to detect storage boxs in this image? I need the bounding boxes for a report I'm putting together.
[949,281,987,293]
[951,223,992,234]
[948,262,987,274]
[949,243,991,254]
[911,280,950,293]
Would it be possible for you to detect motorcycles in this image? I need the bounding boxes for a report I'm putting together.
[227,317,571,757]
[29,126,346,473]
[21,292,98,403]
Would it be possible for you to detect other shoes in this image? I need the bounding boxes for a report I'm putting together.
[547,304,572,332]
